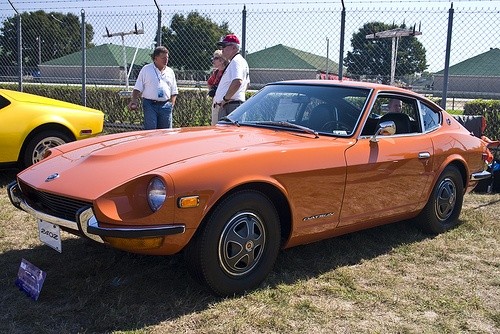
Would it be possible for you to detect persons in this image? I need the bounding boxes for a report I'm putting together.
[128,46,180,131]
[388,99,415,121]
[207,33,250,126]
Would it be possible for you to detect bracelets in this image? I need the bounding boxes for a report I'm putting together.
[223,96,229,103]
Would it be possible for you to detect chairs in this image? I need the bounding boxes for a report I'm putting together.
[307,104,338,131]
[470,135,500,194]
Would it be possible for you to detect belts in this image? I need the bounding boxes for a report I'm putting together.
[143,98,169,105]
[225,101,244,104]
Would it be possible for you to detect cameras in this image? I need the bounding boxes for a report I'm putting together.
[209,84,218,97]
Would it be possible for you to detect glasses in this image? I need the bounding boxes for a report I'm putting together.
[214,57,220,60]
[222,45,231,48]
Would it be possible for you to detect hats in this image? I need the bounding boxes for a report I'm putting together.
[217,34,240,45]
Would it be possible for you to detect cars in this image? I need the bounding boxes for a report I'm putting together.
[0,89,105,174]
[6,79,494,298]
